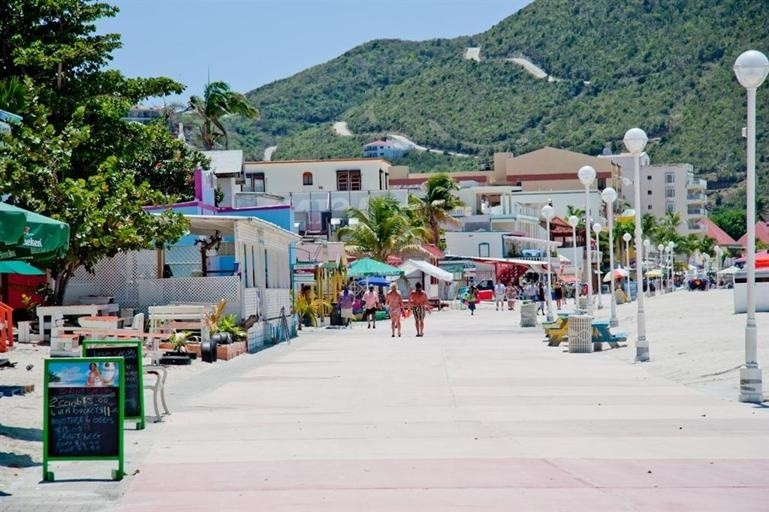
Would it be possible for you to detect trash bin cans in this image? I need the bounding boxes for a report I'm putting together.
[521,304,537,327]
[579,296,587,309]
[330,303,348,325]
[568,315,592,353]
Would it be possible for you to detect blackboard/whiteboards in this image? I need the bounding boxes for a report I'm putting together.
[43,386,124,460]
[82,340,144,419]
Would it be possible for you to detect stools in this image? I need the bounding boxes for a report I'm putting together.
[17,321,36,342]
[51,334,80,351]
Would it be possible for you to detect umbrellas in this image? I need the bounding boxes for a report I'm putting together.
[0,260,49,280]
[0,199,76,264]
[344,256,405,291]
[602,268,633,283]
[357,276,392,287]
[644,268,664,278]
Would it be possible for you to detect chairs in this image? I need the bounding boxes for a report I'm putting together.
[124,313,144,344]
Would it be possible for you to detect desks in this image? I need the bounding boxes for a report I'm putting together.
[554,312,609,351]
[36,304,120,342]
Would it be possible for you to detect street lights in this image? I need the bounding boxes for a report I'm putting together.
[592,222,604,310]
[732,47,767,404]
[540,205,559,324]
[568,215,580,304]
[577,165,596,320]
[622,231,675,303]
[623,127,651,363]
[600,186,620,329]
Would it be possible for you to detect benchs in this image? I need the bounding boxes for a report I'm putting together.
[148,301,219,342]
[541,321,627,348]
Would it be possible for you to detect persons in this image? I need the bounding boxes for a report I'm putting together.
[347,277,356,292]
[301,286,312,306]
[86,362,100,387]
[352,295,361,314]
[612,275,685,304]
[408,282,432,337]
[363,286,379,329]
[464,274,589,316]
[335,288,354,330]
[99,364,114,386]
[104,362,113,370]
[385,281,405,338]
